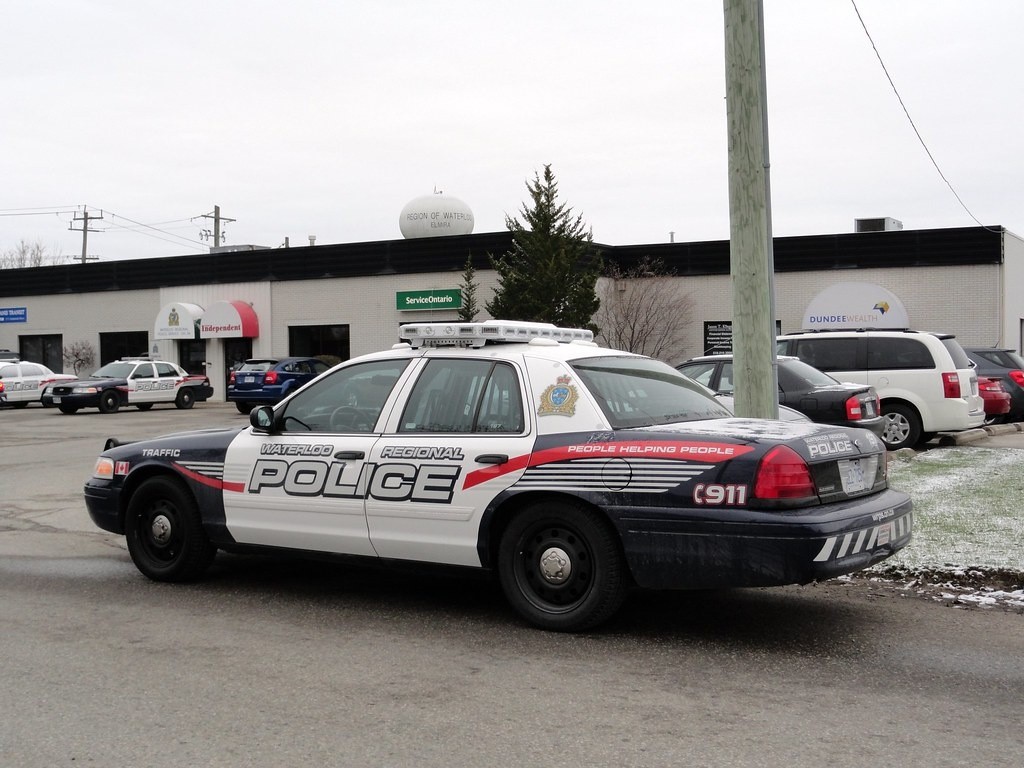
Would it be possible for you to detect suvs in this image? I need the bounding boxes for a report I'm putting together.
[695,327,986,450]
[228,357,331,412]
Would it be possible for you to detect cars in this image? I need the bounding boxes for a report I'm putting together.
[0,349,80,408]
[42,357,214,413]
[963,338,1024,425]
[84,320,914,631]
[673,355,885,439]
[978,376,1012,414]
[687,376,814,424]
